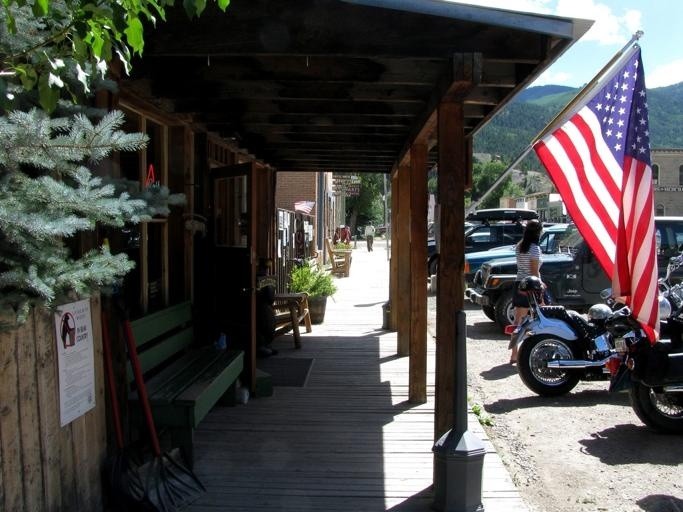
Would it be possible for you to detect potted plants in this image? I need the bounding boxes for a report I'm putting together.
[282,262,334,325]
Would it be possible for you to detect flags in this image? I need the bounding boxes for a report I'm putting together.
[295,200,316,214]
[534,45,659,344]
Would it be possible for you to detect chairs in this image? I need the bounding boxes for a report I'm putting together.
[324,236,353,277]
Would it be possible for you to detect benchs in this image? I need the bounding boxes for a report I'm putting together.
[110,298,246,475]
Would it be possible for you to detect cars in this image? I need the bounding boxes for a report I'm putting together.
[465,224,683,304]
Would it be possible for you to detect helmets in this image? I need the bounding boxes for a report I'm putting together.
[659,297,671,320]
[519,276,545,292]
[588,303,612,321]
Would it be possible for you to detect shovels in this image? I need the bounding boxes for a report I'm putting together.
[116,303,208,512]
[99,306,148,509]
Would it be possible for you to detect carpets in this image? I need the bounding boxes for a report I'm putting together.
[258,356,315,388]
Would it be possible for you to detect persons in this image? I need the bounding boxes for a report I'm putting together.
[364,221,375,251]
[509,219,546,364]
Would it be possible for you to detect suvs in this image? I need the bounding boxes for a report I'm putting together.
[426,221,554,275]
[465,215,683,327]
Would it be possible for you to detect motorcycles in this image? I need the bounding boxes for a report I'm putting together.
[510,256,683,432]
